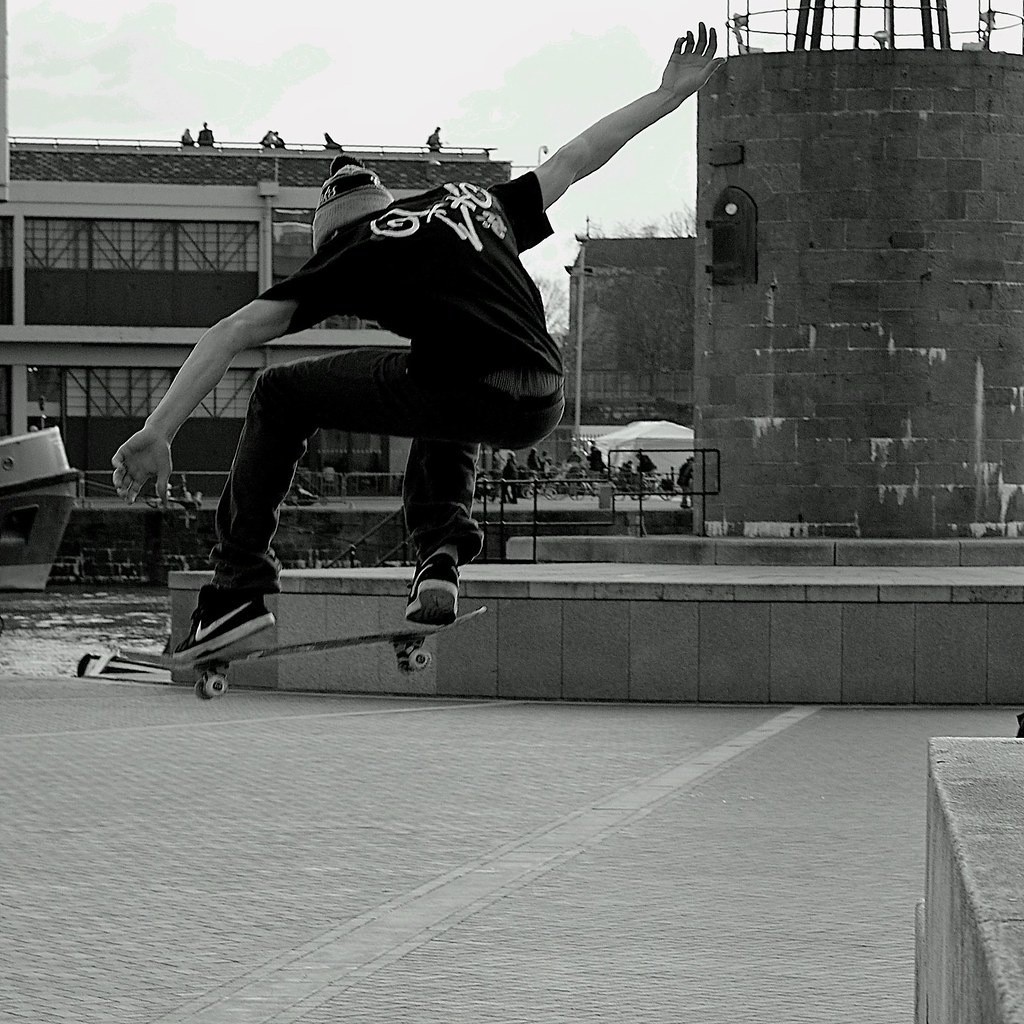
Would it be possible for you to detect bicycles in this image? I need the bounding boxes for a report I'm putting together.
[473,459,677,505]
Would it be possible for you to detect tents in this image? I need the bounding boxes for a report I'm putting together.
[597,421,693,486]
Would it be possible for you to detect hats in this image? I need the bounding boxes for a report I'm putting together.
[313,154,396,254]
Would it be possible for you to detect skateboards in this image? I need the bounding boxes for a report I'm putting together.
[174,605,490,700]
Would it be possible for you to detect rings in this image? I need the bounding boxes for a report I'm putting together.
[121,463,128,472]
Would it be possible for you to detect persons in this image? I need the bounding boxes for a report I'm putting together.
[475,446,606,503]
[622,454,659,493]
[261,131,285,148]
[181,123,214,146]
[426,127,442,153]
[677,457,694,508]
[320,462,335,495]
[113,21,724,662]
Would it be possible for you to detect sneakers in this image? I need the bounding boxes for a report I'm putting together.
[405,553,459,626]
[173,583,275,660]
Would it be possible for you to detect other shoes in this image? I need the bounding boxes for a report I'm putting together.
[499,500,506,503]
[510,499,517,504]
[680,504,690,508]
